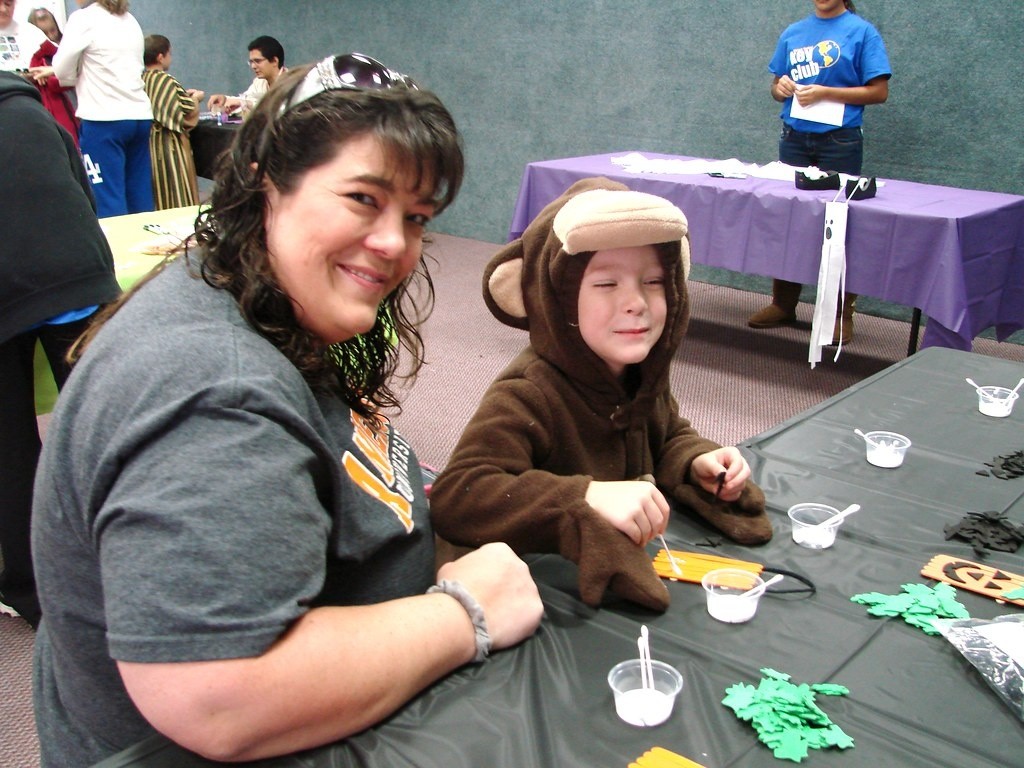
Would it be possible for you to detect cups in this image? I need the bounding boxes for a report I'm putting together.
[702,568,765,622]
[791,501,842,551]
[974,386,1018,418]
[863,430,910,468]
[607,658,683,726]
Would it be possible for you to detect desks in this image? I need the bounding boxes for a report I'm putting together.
[511,150,1023,359]
[185,112,242,184]
[97,202,214,292]
[75,343,1023,768]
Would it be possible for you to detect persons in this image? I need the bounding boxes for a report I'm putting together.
[429,175,771,612]
[30,52,545,768]
[28,7,63,44]
[748,0,892,344]
[207,36,288,122]
[0,72,124,631]
[29,0,157,217]
[142,34,204,209]
[0,0,57,84]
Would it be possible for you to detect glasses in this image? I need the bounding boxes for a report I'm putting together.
[247,56,272,66]
[272,52,422,122]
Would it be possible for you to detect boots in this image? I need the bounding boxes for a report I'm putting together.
[746,278,802,326]
[832,291,858,345]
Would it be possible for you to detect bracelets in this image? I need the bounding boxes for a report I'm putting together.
[423,579,491,667]
